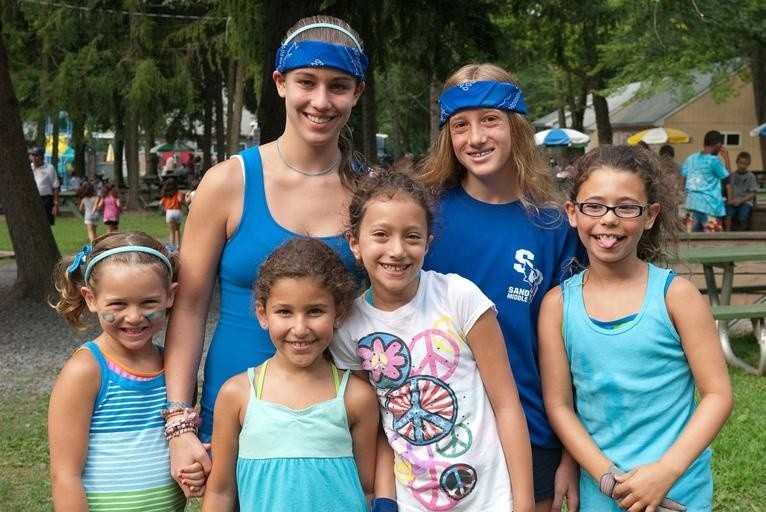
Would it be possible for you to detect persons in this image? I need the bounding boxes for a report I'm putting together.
[28,147,60,225]
[412,64,591,510]
[79,183,101,242]
[657,145,681,176]
[328,172,536,512]
[70,173,106,188]
[679,131,732,233]
[172,153,204,176]
[726,151,757,231]
[164,15,376,498]
[48,233,210,511]
[184,179,198,208]
[200,235,379,512]
[98,183,123,234]
[160,179,182,249]
[538,145,732,511]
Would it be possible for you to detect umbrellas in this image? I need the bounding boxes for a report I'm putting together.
[534,127,591,147]
[150,141,195,156]
[626,127,697,144]
[750,121,766,137]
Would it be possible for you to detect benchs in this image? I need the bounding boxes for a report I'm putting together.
[710,305,766,376]
[664,203,766,240]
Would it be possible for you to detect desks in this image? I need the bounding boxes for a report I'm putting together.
[662,245,763,376]
[57,190,85,222]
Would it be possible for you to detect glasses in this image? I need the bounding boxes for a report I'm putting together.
[573,201,652,219]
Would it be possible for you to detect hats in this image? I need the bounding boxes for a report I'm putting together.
[27,147,44,155]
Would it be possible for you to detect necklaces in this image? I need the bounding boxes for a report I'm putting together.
[276,138,343,175]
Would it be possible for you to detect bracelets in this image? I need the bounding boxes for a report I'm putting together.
[160,406,196,441]
